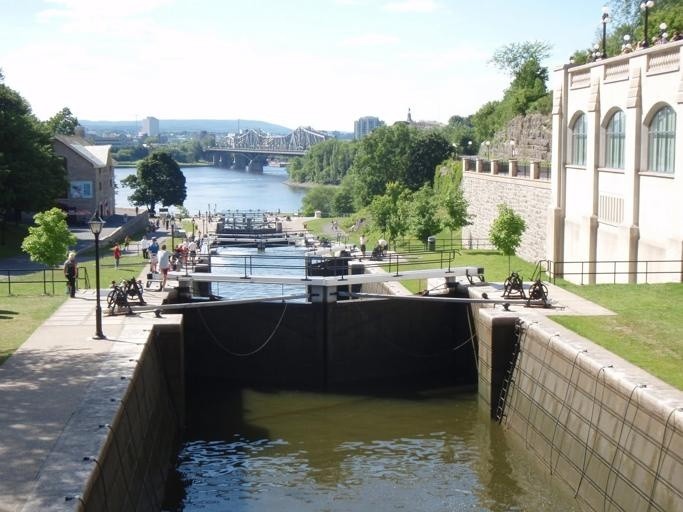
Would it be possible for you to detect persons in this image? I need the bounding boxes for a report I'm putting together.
[114,206,198,288]
[360,234,389,257]
[64,252,77,298]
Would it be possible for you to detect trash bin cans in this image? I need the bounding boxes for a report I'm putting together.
[427,237,435,251]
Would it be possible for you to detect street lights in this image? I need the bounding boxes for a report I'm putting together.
[191,217,201,245]
[167,216,178,253]
[598,0,656,60]
[86,208,106,340]
[450,139,517,159]
